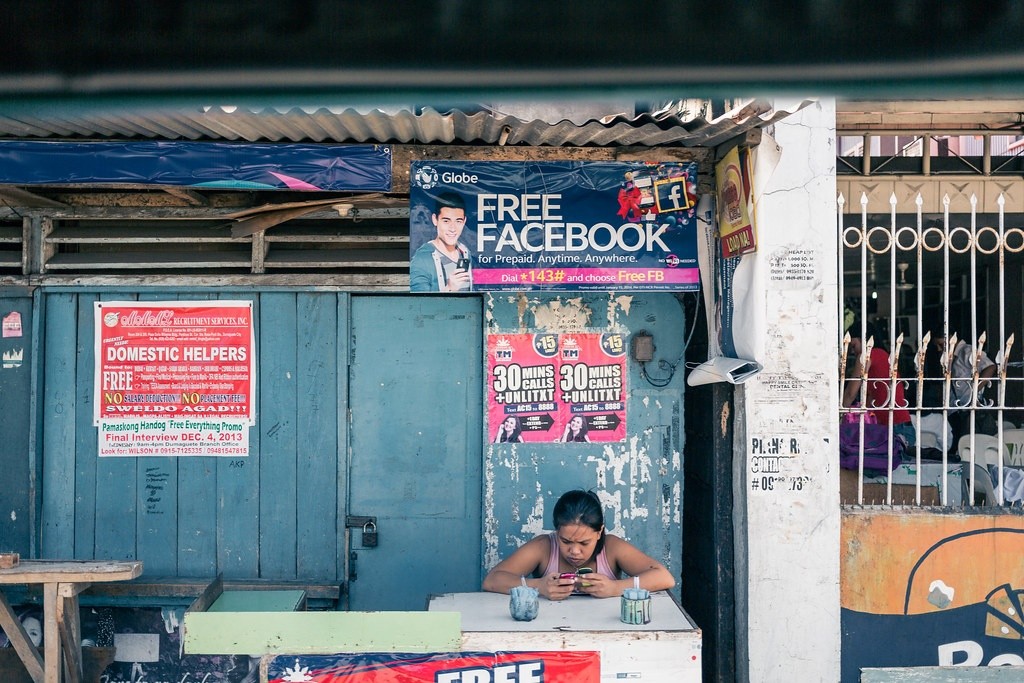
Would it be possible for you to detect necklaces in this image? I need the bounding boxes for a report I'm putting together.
[574,562,588,574]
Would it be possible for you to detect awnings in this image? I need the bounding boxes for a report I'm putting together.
[0,98,820,147]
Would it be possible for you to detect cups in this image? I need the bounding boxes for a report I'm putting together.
[509,586,539,621]
[620,596,651,625]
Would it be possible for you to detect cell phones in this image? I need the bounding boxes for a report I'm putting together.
[457,258,470,274]
[559,573,576,587]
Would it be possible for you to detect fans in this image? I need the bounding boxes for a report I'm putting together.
[875,262,957,291]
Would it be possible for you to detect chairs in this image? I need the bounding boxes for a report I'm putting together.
[959,429,1024,506]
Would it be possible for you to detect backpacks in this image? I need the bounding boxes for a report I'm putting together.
[840,422,907,479]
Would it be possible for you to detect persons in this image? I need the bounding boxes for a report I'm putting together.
[410,191,472,291]
[843,320,996,447]
[493,414,524,443]
[560,414,590,443]
[482,485,676,601]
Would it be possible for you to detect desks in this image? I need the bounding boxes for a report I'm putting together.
[0,560,143,683]
[428,588,704,683]
[986,464,1024,506]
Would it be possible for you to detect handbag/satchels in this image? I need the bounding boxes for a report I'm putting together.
[947,408,998,442]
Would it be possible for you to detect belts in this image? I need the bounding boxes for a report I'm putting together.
[904,421,913,427]
